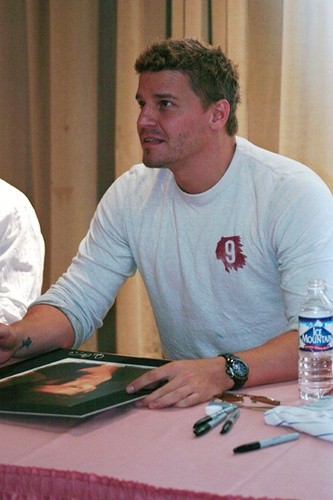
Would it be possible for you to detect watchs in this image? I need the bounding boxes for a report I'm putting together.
[219,352,251,391]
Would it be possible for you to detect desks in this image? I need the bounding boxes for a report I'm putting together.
[0,381,332,500]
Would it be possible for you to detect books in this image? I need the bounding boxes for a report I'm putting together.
[1,346,175,419]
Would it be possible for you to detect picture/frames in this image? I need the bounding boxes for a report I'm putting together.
[1,348,173,417]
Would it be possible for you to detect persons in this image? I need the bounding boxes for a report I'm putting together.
[2,178,45,329]
[1,35,331,409]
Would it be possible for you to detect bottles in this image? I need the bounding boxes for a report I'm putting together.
[298,279,333,402]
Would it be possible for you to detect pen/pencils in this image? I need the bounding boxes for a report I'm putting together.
[193,404,300,453]
